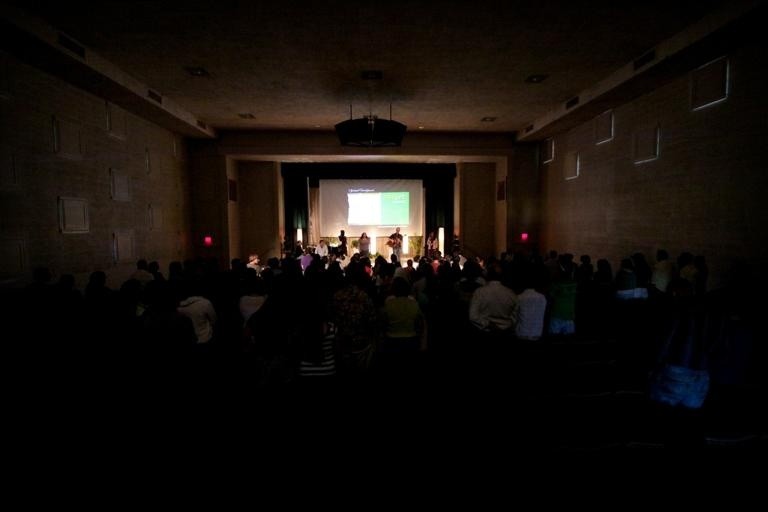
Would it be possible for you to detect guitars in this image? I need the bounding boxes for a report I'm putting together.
[387,234,407,247]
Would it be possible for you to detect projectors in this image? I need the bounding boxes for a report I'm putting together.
[334,118,407,148]
